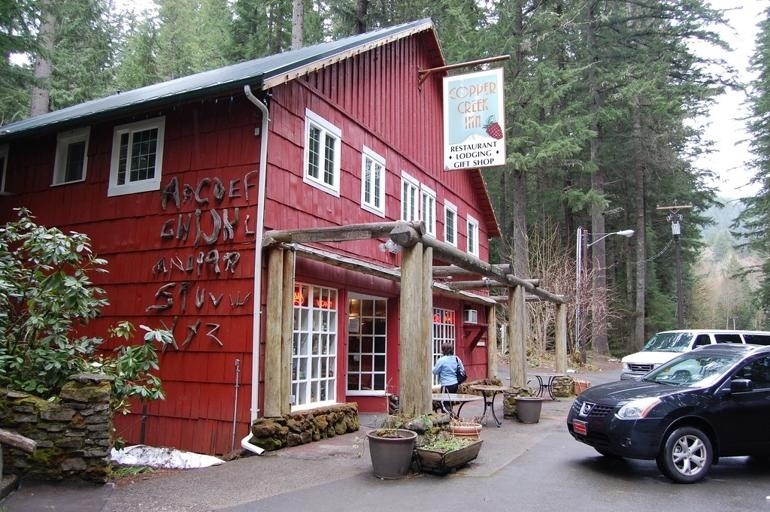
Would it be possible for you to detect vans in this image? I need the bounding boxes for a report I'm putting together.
[620,329,770,380]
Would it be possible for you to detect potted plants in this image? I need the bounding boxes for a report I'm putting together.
[416,438,483,476]
[366,428,417,480]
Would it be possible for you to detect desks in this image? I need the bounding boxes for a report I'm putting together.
[432,393,482,419]
[528,373,565,402]
[470,385,507,428]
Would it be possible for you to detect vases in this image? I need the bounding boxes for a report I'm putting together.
[575,381,591,395]
[449,423,482,439]
[513,397,544,424]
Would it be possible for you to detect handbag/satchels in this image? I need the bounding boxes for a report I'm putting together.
[456,357,468,385]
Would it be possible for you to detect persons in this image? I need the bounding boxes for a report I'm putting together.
[432,342,465,413]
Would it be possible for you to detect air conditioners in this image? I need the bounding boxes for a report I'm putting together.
[464,309,478,324]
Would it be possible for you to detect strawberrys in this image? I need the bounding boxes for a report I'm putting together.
[483,116,503,141]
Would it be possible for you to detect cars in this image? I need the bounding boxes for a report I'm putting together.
[567,343,770,484]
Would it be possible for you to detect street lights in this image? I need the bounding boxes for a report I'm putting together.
[581,230,635,363]
